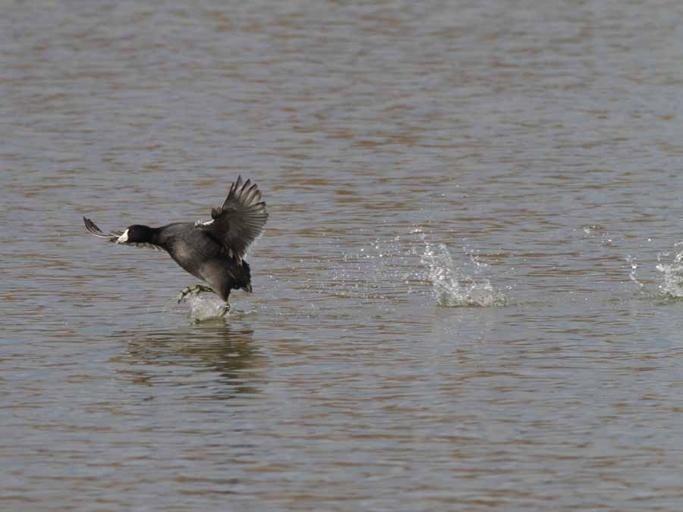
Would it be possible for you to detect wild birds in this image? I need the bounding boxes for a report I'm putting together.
[80,175,269,320]
